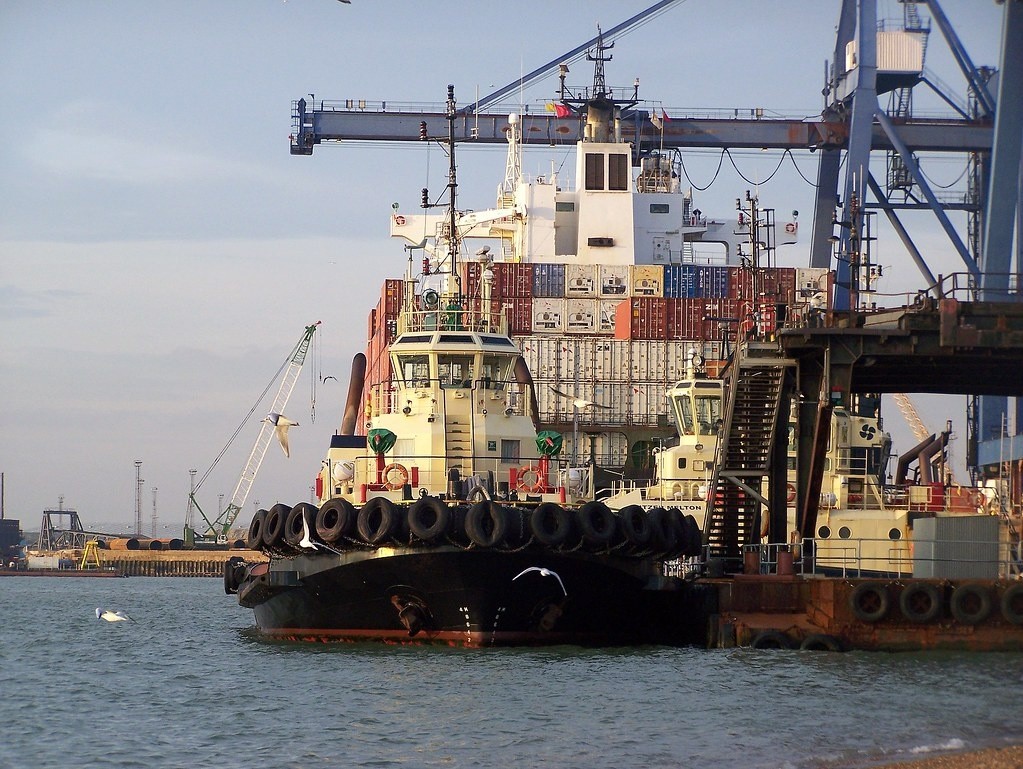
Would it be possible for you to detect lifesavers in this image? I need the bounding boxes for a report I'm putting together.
[752,632,841,652]
[381,463,408,490]
[357,497,399,543]
[682,514,702,556]
[849,581,1023,626]
[648,507,675,550]
[579,500,616,542]
[965,490,985,507]
[285,502,320,547]
[516,465,543,492]
[464,500,505,546]
[408,495,449,540]
[619,505,651,544]
[263,504,293,546]
[531,502,569,544]
[224,555,244,594]
[315,498,354,542]
[668,508,689,546]
[787,484,796,502]
[248,509,270,551]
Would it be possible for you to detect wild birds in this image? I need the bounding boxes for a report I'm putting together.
[260,412,301,457]
[322,376,338,384]
[95,608,137,624]
[512,566,567,596]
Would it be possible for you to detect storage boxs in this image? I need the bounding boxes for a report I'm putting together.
[348,263,829,434]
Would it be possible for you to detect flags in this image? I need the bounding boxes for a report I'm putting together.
[546,103,556,112]
[555,104,572,118]
[663,110,670,122]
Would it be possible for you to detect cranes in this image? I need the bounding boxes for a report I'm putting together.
[181,320,322,552]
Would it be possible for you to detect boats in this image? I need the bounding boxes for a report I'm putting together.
[222,0,1023,657]
